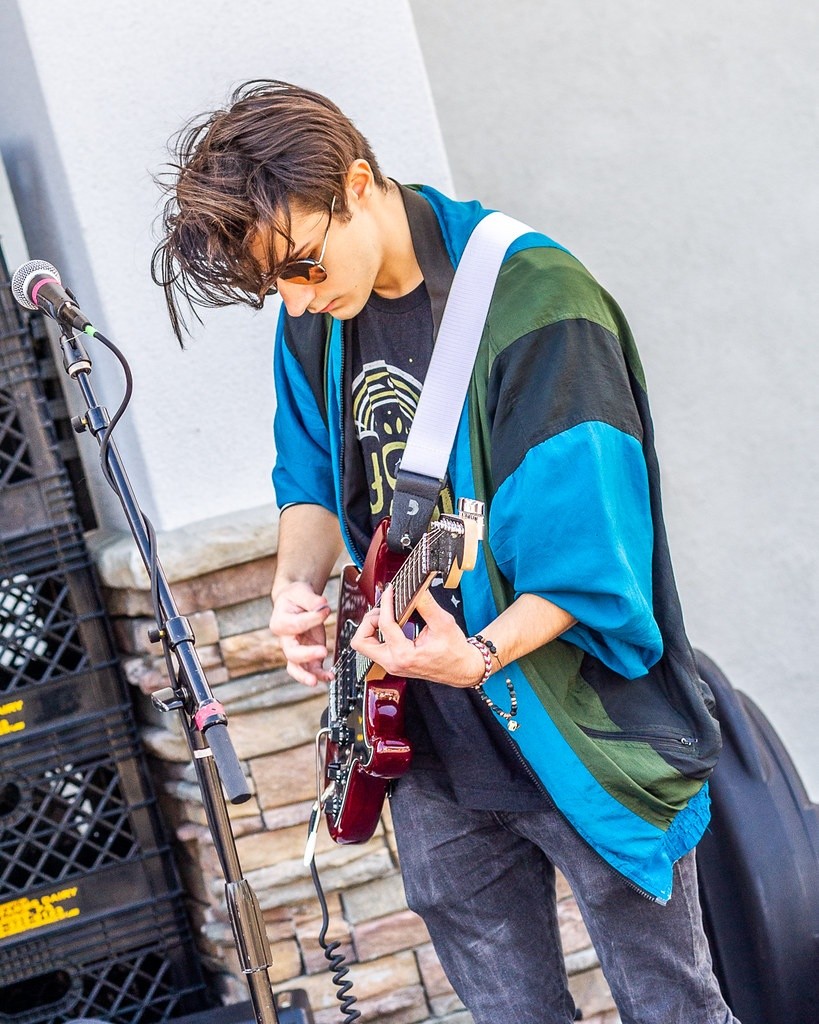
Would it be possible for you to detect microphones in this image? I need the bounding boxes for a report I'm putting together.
[11,259,92,333]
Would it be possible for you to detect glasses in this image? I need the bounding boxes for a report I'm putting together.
[259,196,336,295]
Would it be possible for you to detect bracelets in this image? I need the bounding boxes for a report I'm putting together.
[466,635,520,720]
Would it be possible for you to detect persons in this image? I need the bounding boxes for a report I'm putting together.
[149,77,739,1024]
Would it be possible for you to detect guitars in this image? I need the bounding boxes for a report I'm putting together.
[311,513,480,848]
